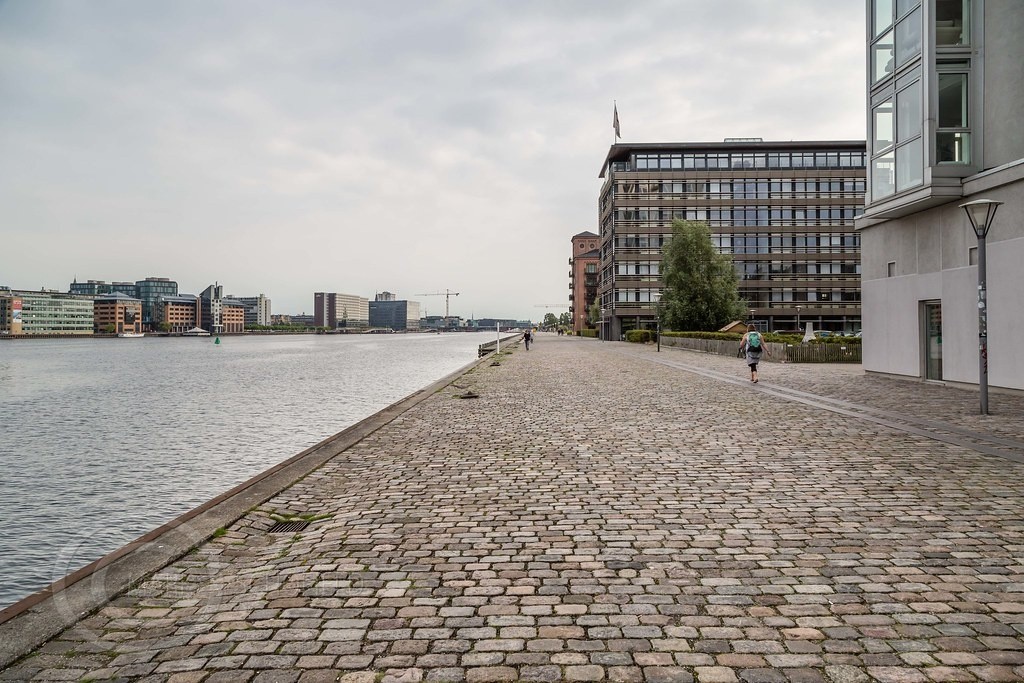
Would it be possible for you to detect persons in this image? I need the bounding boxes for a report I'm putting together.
[524,330,530,350]
[739,324,771,384]
[530,331,534,343]
[558,328,563,336]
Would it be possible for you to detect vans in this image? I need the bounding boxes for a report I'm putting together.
[773,329,861,338]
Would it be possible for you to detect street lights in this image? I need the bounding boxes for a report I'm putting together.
[579,314,584,339]
[958,198,1006,414]
[750,309,757,324]
[795,305,802,329]
[600,309,607,343]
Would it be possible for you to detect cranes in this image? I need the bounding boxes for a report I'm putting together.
[413,288,459,328]
[534,303,571,313]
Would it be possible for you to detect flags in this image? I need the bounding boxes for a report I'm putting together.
[613,104,621,138]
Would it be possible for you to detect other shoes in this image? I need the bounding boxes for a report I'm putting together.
[750,378,759,383]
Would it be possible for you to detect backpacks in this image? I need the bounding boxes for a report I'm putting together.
[747,332,760,347]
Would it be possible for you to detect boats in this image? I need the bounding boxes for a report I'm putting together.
[118,330,146,338]
